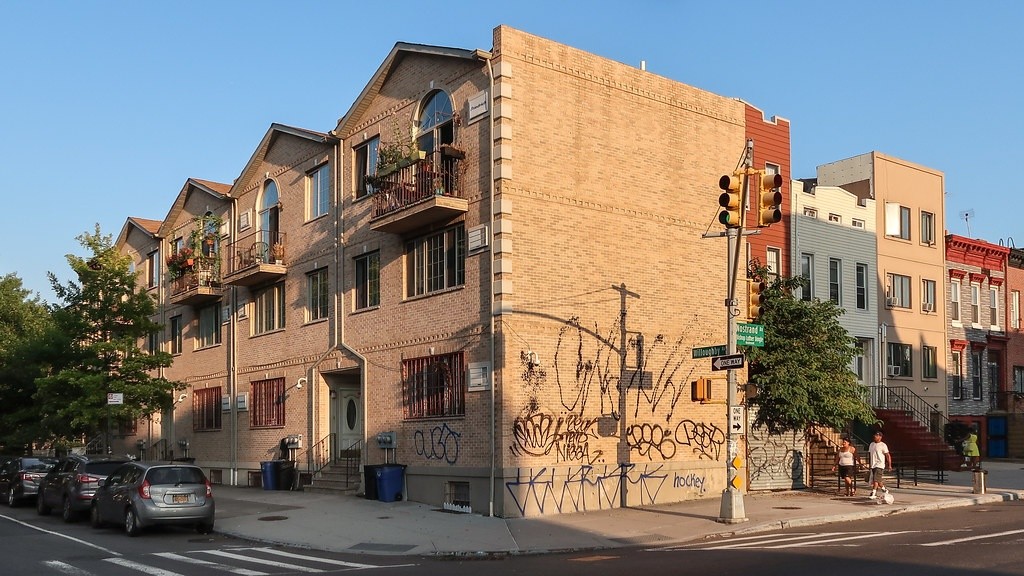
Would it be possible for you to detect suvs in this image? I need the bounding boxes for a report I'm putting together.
[36,456,134,525]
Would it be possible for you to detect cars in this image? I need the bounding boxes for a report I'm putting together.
[90,461,215,537]
[0,457,61,508]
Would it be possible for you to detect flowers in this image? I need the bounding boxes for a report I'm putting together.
[271,242,285,259]
[167,247,193,268]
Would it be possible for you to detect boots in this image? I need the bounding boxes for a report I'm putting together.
[868,491,875,499]
[881,486,889,494]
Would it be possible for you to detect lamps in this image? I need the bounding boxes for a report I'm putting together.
[178,393,188,403]
[528,350,540,366]
[297,375,309,389]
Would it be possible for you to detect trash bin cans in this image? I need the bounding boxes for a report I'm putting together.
[373,463,407,502]
[364,465,381,499]
[260,461,285,490]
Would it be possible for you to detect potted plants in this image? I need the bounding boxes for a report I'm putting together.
[375,112,459,217]
[204,233,214,244]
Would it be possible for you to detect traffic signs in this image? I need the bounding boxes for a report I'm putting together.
[711,353,745,371]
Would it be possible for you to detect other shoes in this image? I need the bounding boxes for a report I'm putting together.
[844,492,848,496]
[850,492,854,496]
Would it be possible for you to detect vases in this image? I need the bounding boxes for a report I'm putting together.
[275,259,283,267]
[179,258,193,268]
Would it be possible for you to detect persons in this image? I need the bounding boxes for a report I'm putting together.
[832,437,862,496]
[867,431,892,500]
[955,425,979,466]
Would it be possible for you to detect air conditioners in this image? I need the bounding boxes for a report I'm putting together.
[888,365,900,376]
[922,303,933,312]
[886,297,898,306]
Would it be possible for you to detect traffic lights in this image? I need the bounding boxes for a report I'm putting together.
[749,281,766,318]
[759,173,782,227]
[718,174,741,227]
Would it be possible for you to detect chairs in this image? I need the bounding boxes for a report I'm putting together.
[248,242,269,267]
[237,251,254,270]
